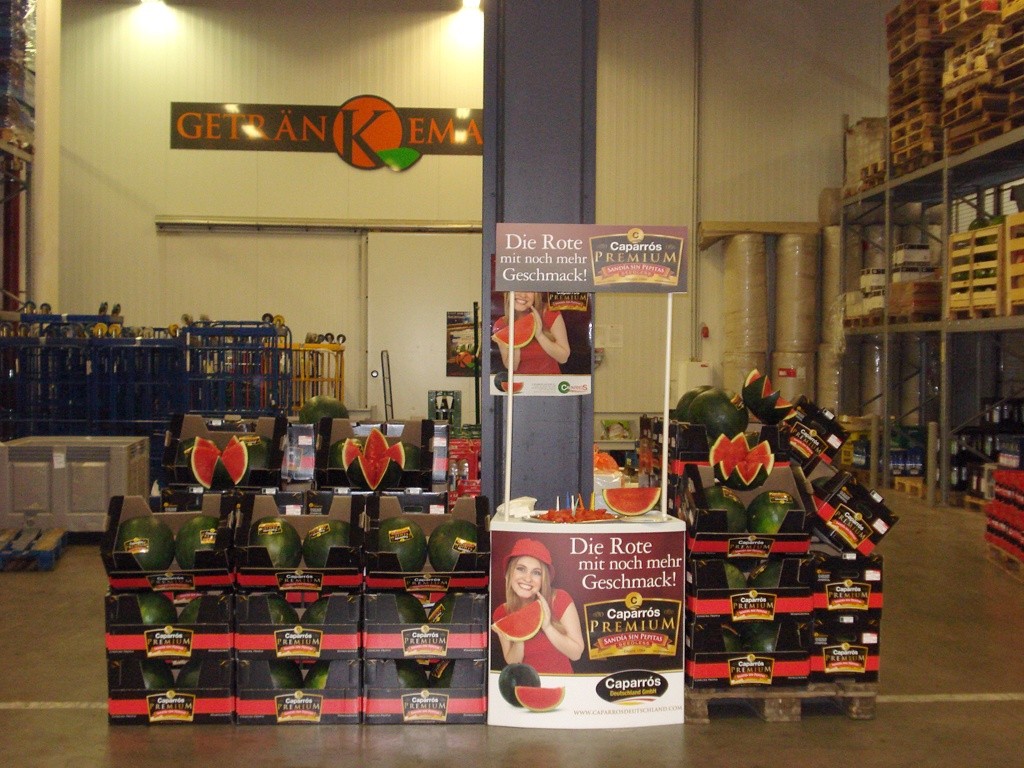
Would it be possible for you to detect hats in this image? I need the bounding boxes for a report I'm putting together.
[502,539,555,585]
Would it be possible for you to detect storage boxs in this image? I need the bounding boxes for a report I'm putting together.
[844,244,944,327]
[101,412,1024,728]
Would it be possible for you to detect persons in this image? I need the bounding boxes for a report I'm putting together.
[492,539,585,673]
[493,291,570,375]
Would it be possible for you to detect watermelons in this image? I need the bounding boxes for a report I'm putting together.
[176,434,275,489]
[493,600,543,641]
[538,507,615,523]
[300,394,349,427]
[498,662,564,712]
[327,427,421,491]
[675,368,799,653]
[113,515,481,689]
[603,487,662,516]
[494,312,537,348]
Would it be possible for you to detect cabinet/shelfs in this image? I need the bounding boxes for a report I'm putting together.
[843,109,1024,504]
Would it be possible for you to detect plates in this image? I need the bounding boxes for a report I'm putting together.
[521,515,619,523]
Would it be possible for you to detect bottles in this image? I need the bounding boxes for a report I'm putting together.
[440,395,449,420]
[435,399,439,420]
[458,458,469,485]
[447,463,458,492]
[449,398,454,425]
